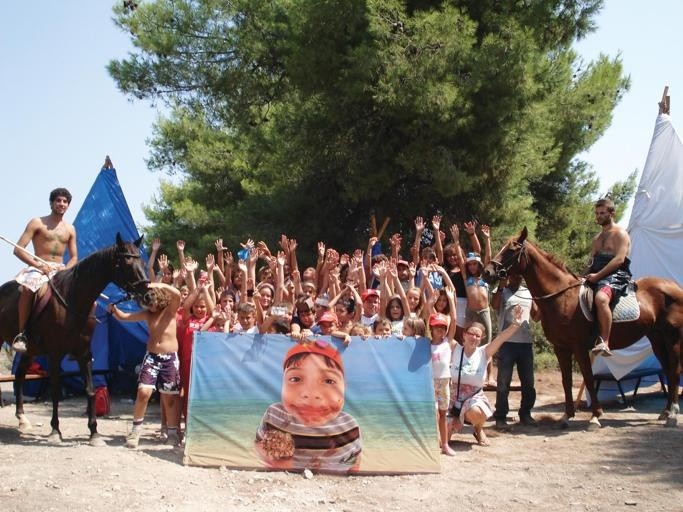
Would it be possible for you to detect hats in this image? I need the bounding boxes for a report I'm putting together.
[284,341,344,374]
[361,289,379,301]
[317,311,338,326]
[397,260,408,267]
[466,252,485,269]
[295,296,313,313]
[429,313,448,327]
[422,247,436,258]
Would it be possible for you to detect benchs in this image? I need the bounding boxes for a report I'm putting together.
[482,385,522,392]
[0,369,118,406]
[594,368,668,412]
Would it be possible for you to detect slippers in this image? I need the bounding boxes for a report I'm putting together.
[473,431,488,447]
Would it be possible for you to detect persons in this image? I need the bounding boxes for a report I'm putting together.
[492,262,541,426]
[109,283,181,450]
[585,199,632,357]
[147,214,522,457]
[14,188,78,352]
[254,339,361,475]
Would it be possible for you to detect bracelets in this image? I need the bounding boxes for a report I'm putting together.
[157,271,165,277]
[469,233,474,236]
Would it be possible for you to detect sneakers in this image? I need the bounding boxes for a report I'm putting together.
[495,419,508,431]
[13,340,27,352]
[441,445,454,457]
[127,431,140,447]
[519,416,537,428]
[160,425,179,451]
[591,343,612,356]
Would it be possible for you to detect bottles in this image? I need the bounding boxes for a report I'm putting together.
[120,399,137,404]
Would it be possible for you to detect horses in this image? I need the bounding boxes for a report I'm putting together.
[0,231,159,448]
[481,225,683,431]
